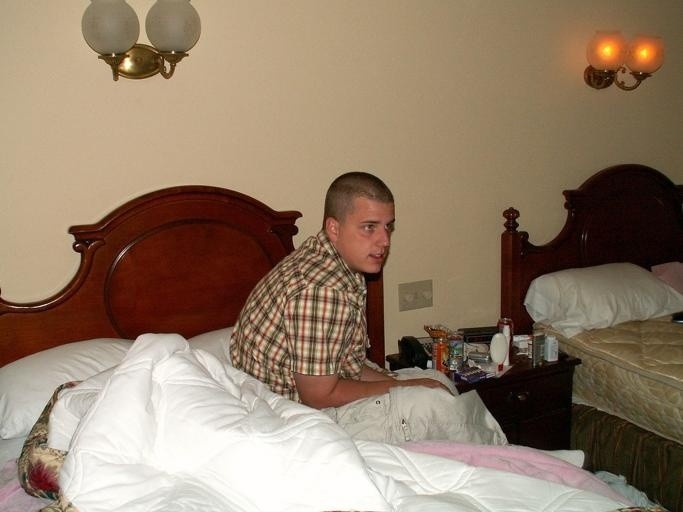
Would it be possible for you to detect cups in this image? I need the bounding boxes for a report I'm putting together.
[447,330,463,370]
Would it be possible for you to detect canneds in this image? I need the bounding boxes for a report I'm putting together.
[497,318,514,365]
[431,338,449,375]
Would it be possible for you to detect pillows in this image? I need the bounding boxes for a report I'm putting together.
[522,258,682,341]
[183,324,242,366]
[648,254,683,301]
[1,334,142,442]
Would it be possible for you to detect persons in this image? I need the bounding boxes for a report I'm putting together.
[229,171,455,409]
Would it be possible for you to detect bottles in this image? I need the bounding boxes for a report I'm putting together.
[527,339,532,359]
[543,335,558,361]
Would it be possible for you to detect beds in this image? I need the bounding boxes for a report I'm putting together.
[0,187,666,512]
[498,165,682,510]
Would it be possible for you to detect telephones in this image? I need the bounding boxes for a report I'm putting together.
[398,336,435,371]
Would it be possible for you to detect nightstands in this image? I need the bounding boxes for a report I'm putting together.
[382,351,581,456]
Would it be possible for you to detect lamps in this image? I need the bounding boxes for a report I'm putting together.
[80,0,201,85]
[582,26,663,93]
